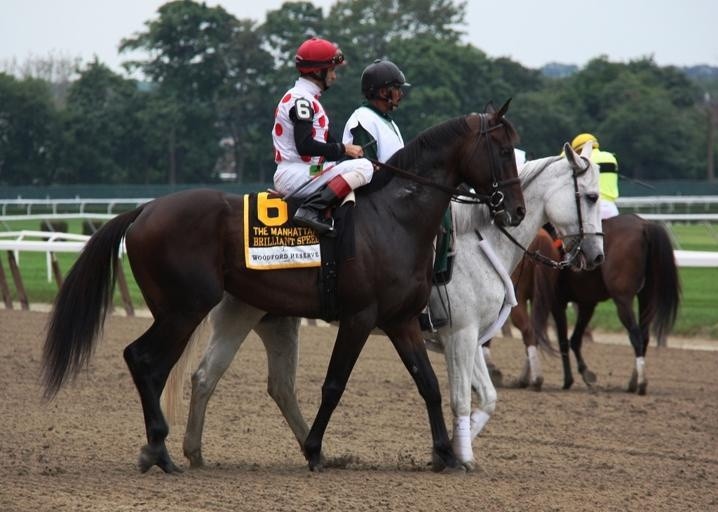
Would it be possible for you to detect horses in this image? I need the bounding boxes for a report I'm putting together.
[35,94,526,476]
[541,210,682,396]
[481,226,562,393]
[159,139,605,472]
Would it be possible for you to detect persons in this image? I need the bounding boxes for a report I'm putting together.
[272,37,375,238]
[572,133,620,220]
[341,58,449,333]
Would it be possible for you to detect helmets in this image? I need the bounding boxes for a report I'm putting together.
[360,56,412,96]
[293,38,347,74]
[569,133,600,152]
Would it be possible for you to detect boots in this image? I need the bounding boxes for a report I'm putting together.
[292,174,353,237]
